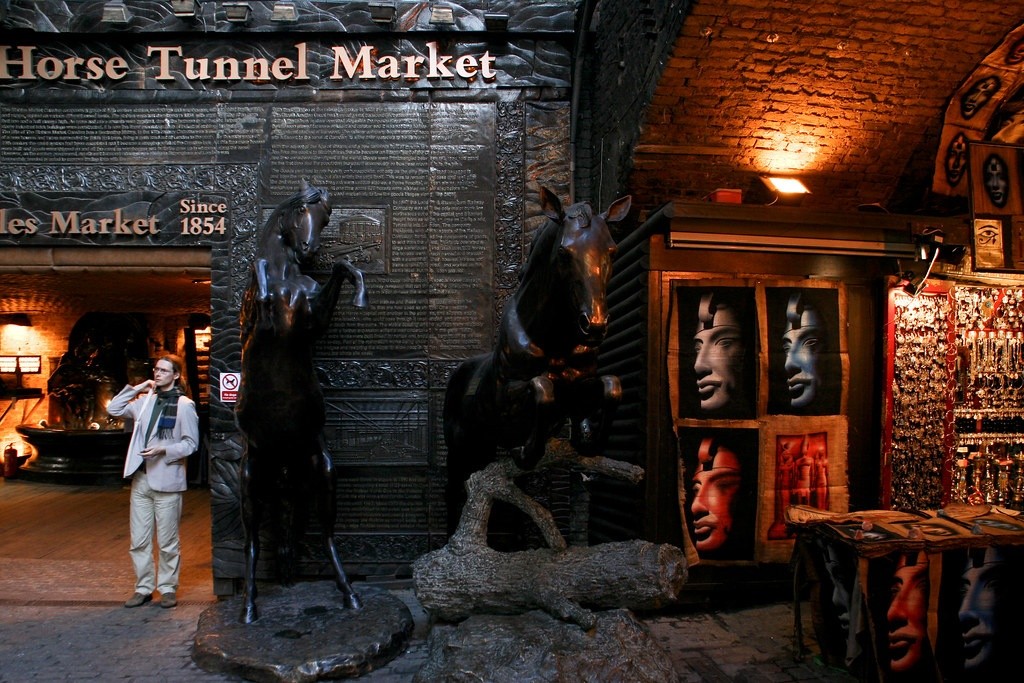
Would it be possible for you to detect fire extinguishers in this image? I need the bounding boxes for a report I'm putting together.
[3,440,20,481]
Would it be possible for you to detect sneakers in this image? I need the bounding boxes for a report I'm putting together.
[160,592,177,608]
[125,591,153,608]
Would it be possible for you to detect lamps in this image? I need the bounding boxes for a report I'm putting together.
[99,0,511,25]
[753,175,813,206]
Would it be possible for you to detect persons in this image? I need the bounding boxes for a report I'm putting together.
[105,353,200,609]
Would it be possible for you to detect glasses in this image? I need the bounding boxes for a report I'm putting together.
[152,367,173,375]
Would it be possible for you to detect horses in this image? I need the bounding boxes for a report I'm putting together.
[444,186,632,536]
[235,179,366,625]
